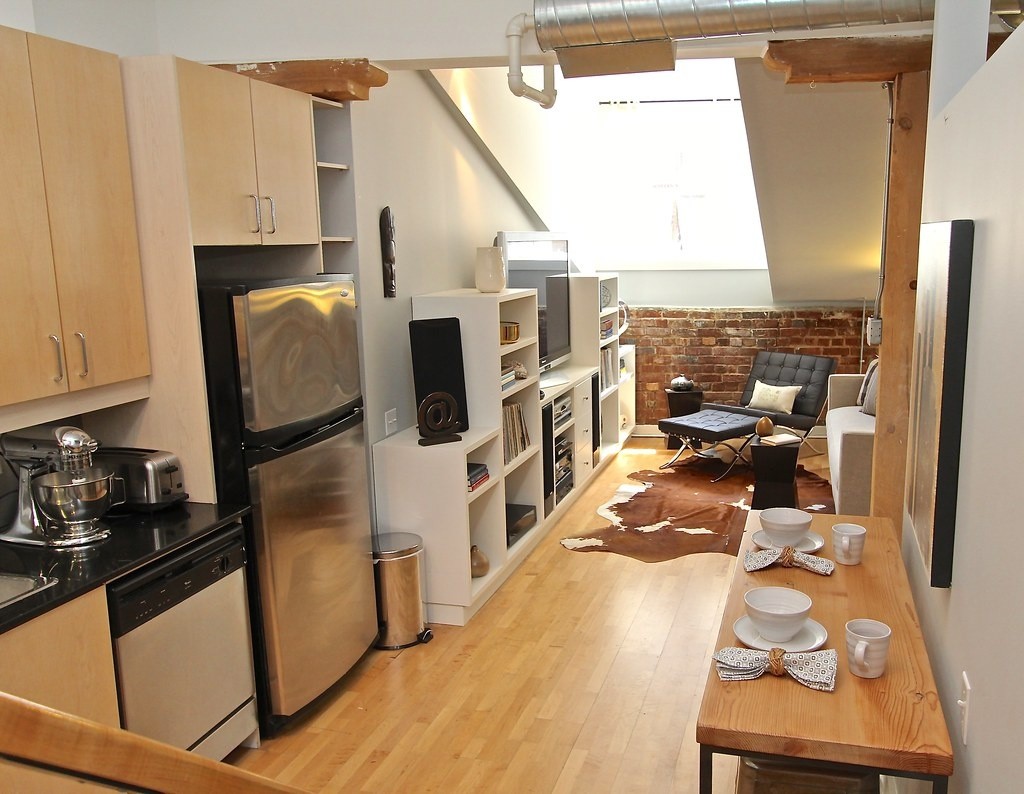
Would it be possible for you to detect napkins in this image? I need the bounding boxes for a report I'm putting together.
[743,548,834,576]
[711,647,838,692]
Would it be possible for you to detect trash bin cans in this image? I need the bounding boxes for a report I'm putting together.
[370,531,433,652]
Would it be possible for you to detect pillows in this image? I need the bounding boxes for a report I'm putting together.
[745,380,803,415]
[856,359,878,417]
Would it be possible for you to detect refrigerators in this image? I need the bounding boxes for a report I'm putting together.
[191,271,381,738]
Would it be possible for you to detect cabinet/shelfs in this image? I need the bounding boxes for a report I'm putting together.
[0,25,152,434]
[372,273,635,626]
[0,583,120,728]
[175,57,354,278]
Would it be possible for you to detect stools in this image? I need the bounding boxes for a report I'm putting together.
[658,409,762,482]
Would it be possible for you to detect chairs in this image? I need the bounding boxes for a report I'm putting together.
[701,351,839,455]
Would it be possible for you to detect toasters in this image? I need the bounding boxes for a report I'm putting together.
[95,446,189,526]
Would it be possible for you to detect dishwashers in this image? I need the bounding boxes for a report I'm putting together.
[106,521,261,765]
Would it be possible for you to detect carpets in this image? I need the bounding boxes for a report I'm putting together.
[559,449,836,563]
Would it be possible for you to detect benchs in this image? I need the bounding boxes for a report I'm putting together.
[826,374,876,516]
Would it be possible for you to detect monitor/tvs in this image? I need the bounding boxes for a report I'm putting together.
[492,231,573,374]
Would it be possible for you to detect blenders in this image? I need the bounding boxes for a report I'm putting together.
[1,427,115,546]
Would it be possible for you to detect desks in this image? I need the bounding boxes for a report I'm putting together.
[664,389,702,450]
[749,427,807,509]
[696,510,954,794]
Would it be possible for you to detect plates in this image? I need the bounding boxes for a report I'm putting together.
[733,615,828,653]
[751,529,824,553]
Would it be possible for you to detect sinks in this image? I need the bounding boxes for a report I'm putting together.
[0,570,58,610]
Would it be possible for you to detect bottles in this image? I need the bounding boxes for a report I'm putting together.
[475,246,506,293]
[471,545,490,577]
[671,374,694,391]
[756,415,774,440]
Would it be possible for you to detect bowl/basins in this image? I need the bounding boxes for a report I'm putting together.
[501,321,520,344]
[759,507,813,547]
[744,586,813,642]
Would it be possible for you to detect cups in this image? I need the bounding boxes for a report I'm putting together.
[845,619,892,679]
[832,523,867,566]
